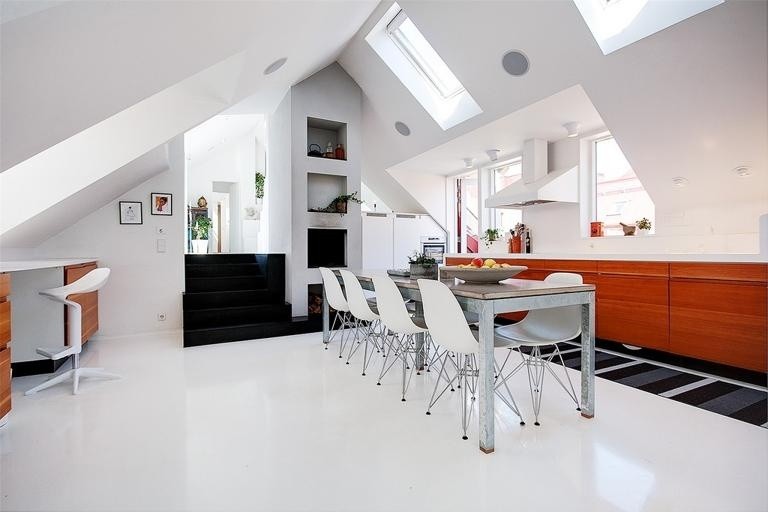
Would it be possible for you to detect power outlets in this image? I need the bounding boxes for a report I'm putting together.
[159,312,165,321]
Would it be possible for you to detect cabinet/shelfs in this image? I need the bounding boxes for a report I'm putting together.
[598,260,669,352]
[188,201,208,253]
[669,261,768,374]
[544,259,598,339]
[496,258,544,321]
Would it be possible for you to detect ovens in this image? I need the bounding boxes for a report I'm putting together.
[421,234,446,264]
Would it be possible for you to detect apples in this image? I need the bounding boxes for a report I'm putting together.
[471,258,483,268]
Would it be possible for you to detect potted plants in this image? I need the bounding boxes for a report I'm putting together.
[255,170,266,205]
[480,228,499,248]
[317,190,364,218]
[407,251,438,281]
[191,214,213,254]
[636,217,652,236]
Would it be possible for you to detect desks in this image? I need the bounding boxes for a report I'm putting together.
[0,257,98,428]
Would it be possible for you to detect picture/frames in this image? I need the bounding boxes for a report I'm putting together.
[151,192,172,216]
[119,201,143,224]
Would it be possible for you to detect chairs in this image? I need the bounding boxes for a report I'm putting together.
[495,272,584,426]
[319,267,359,358]
[417,278,525,441]
[24,267,122,395]
[339,270,380,376]
[370,273,429,401]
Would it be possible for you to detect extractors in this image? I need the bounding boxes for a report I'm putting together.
[485,167,580,209]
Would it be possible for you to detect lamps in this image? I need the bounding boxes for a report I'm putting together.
[485,148,500,161]
[563,121,578,138]
[463,157,472,168]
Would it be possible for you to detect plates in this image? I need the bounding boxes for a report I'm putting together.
[439,266,528,284]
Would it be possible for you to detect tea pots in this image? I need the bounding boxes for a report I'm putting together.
[308,143,325,157]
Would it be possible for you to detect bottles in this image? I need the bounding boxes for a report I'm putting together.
[519,224,525,253]
[525,228,530,253]
